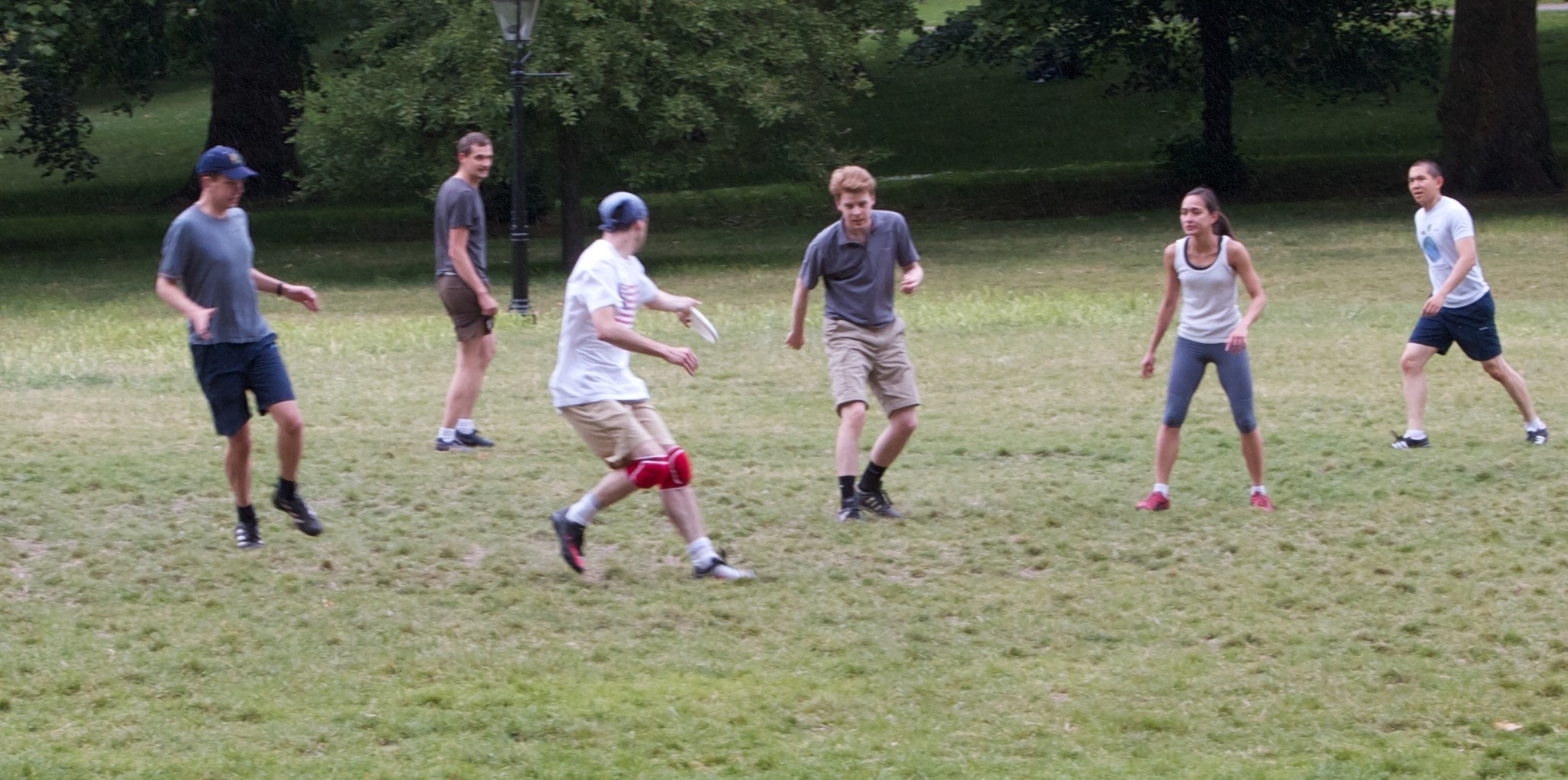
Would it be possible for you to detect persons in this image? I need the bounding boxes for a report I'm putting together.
[1135,188,1275,511]
[156,145,323,550]
[783,166,924,521]
[1389,161,1548,451]
[434,133,498,451]
[550,191,754,582]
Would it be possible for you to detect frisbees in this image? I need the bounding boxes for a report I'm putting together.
[686,305,721,346]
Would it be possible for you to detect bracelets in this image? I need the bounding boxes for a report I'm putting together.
[276,283,283,297]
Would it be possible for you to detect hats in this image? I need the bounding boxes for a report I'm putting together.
[195,145,260,180]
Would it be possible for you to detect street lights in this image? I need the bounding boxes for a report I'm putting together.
[493,0,553,315]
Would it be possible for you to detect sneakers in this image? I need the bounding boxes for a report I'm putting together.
[1389,430,1430,448]
[857,481,901,519]
[1136,492,1171,511]
[693,558,755,580]
[235,518,264,549]
[838,498,863,522]
[435,437,464,452]
[551,507,585,574]
[1251,491,1272,512]
[455,428,494,446]
[1527,425,1547,445]
[273,491,321,536]
